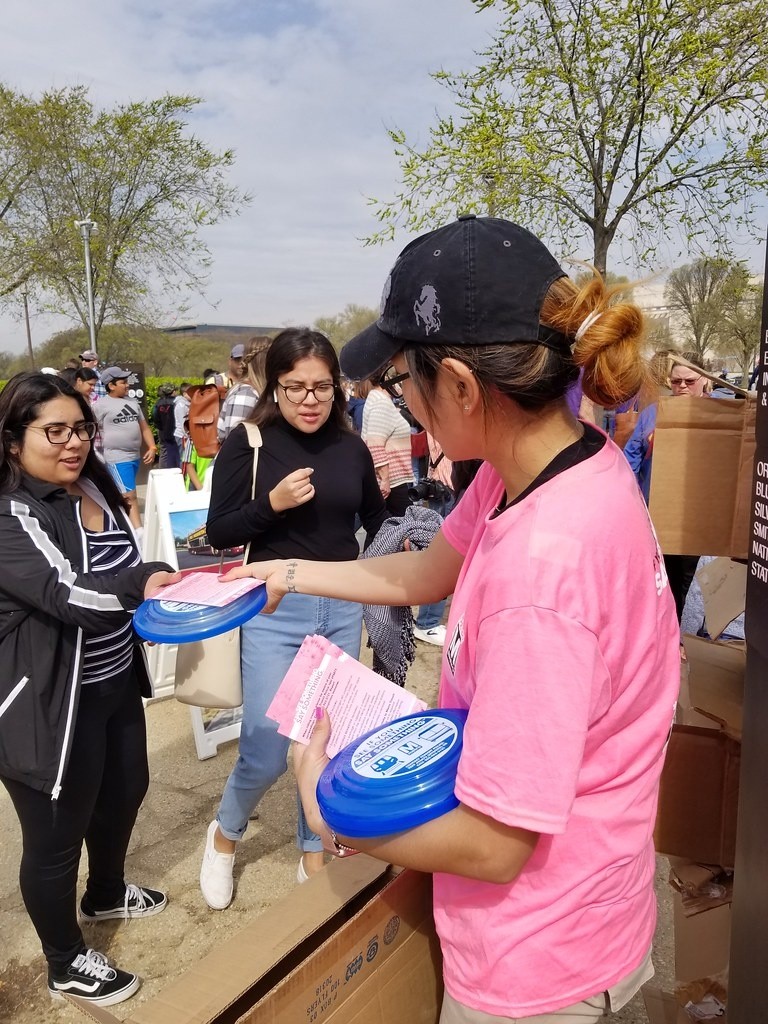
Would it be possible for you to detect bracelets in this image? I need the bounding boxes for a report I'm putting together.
[320,826,362,860]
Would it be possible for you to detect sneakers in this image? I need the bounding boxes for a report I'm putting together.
[296,854,310,885]
[200,820,237,910]
[45,950,142,1009]
[414,625,447,647]
[79,885,168,921]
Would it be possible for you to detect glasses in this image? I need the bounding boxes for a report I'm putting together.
[20,422,97,445]
[670,375,702,385]
[81,358,95,362]
[275,381,341,404]
[378,364,422,399]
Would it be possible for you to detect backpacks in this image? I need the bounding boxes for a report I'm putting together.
[187,377,262,459]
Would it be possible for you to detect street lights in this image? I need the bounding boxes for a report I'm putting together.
[74,219,101,353]
[20,289,35,372]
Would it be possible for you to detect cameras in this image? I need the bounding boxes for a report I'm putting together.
[407,477,451,502]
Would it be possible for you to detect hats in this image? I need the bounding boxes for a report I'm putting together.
[229,344,246,358]
[100,367,133,386]
[161,383,177,394]
[78,349,99,362]
[339,212,574,382]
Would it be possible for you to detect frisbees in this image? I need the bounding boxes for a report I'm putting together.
[313,706,473,838]
[131,584,269,645]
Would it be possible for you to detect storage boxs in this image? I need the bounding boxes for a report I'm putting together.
[642,388,757,1023]
[59,853,443,1024]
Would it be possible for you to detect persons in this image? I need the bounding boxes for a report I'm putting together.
[563,341,741,642]
[151,335,273,493]
[43,345,158,542]
[222,214,685,1024]
[1,370,184,1006]
[336,354,487,648]
[201,324,413,917]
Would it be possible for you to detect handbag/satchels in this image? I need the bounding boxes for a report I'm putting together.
[411,429,431,458]
[614,396,643,449]
[171,627,242,710]
